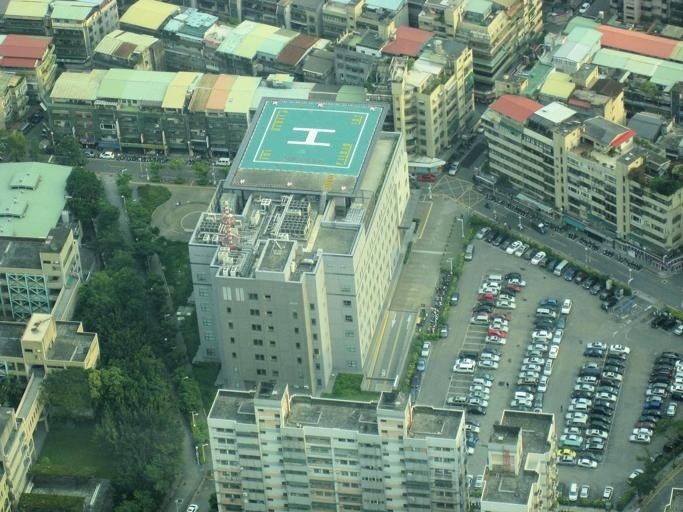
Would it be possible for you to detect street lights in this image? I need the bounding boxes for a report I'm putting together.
[457,218,464,238]
[192,413,198,425]
[201,443,208,462]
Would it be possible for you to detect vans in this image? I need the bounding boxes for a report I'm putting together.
[17,122,31,134]
[215,158,230,166]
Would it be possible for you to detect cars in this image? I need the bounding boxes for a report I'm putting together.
[448,161,459,176]
[83,149,95,158]
[477,125,483,134]
[186,503,198,511]
[579,3,590,14]
[409,173,415,180]
[98,151,114,159]
[416,173,436,182]
[416,221,683,500]
[30,111,42,123]
